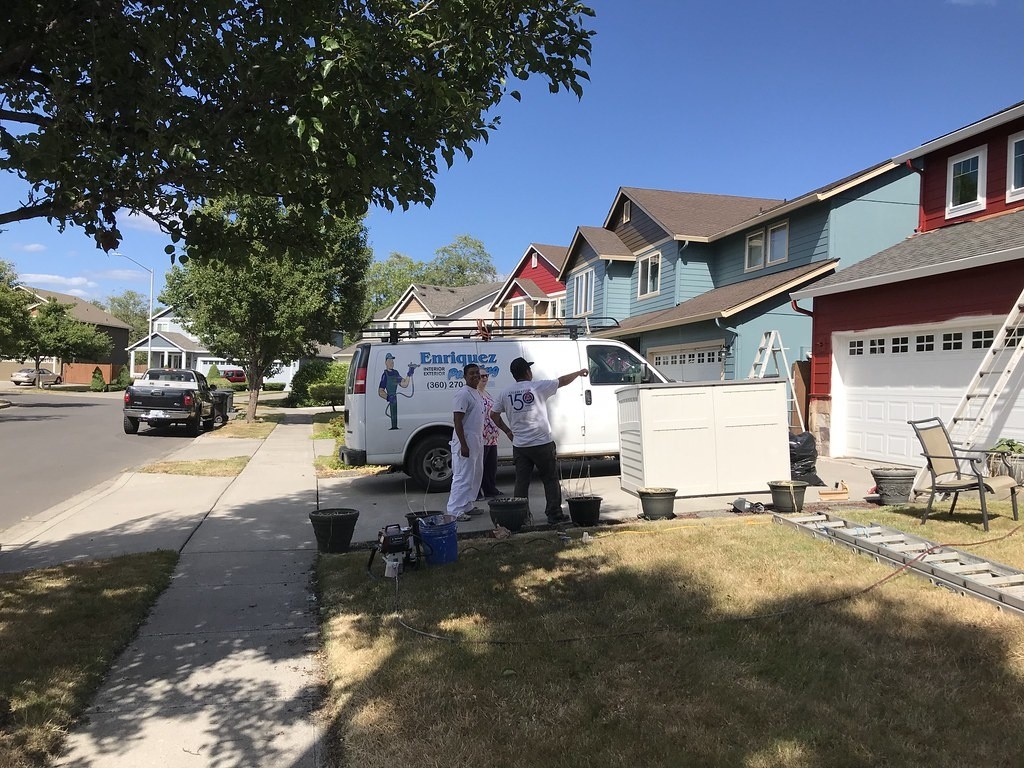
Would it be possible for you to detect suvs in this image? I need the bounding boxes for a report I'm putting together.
[219,370,246,383]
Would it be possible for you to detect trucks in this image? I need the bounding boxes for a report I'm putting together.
[339,317,690,494]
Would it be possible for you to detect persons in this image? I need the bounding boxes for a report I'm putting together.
[490,356,588,523]
[476,368,503,501]
[446,364,484,521]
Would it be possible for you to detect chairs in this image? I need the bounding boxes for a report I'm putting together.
[907,417,1018,532]
[149,373,160,379]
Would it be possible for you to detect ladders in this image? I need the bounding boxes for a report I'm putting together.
[748,330,806,434]
[909,288,1024,502]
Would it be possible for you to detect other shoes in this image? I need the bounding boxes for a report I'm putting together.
[478,497,486,501]
[467,507,484,514]
[548,514,569,523]
[496,490,503,495]
[457,514,471,521]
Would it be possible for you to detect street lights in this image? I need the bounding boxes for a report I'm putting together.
[111,253,154,369]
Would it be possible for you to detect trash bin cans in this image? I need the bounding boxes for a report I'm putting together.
[211,391,229,423]
[218,389,238,413]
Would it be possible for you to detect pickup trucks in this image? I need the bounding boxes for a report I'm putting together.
[123,368,217,437]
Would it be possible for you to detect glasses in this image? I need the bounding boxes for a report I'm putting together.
[481,374,489,378]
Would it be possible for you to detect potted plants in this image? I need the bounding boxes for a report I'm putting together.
[985,438,1024,486]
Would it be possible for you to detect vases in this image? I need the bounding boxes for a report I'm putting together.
[488,499,528,531]
[310,508,359,553]
[768,480,808,512]
[637,488,678,519]
[405,511,444,546]
[871,468,917,505]
[565,497,602,525]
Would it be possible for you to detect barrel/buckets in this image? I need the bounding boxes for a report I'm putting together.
[418,514,459,564]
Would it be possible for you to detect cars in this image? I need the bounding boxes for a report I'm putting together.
[10,368,63,386]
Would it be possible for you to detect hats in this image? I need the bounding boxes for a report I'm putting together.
[510,357,534,373]
